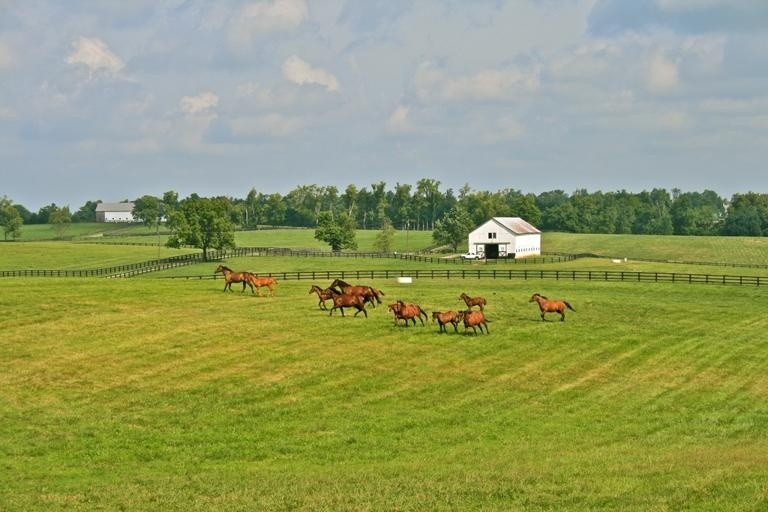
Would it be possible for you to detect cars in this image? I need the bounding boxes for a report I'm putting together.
[461,252,480,260]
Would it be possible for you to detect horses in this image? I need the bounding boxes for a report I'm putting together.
[528,292,576,322]
[458,293,488,313]
[308,278,387,318]
[244,270,280,297]
[457,308,493,336]
[431,310,458,333]
[213,263,255,295]
[387,298,429,328]
